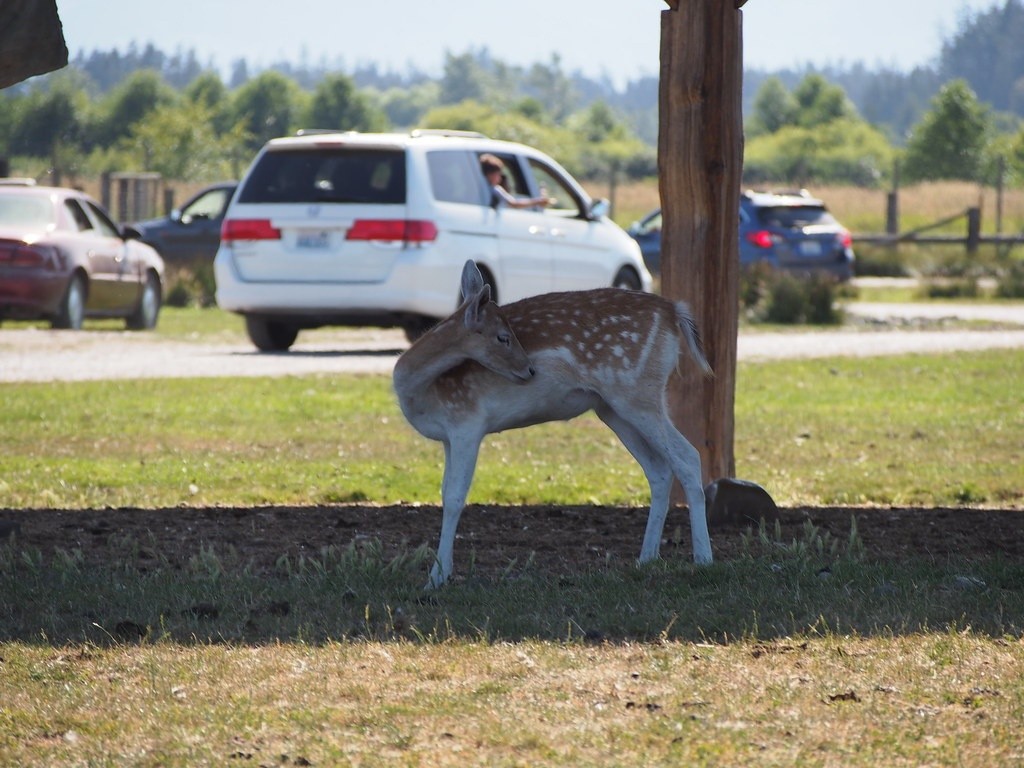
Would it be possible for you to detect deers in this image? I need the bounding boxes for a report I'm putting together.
[391,259,720,592]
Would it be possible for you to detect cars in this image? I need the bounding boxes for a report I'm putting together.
[119,181,237,263]
[0,177,165,331]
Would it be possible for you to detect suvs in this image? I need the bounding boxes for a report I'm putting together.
[626,188,857,320]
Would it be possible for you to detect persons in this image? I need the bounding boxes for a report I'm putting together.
[480,154,550,211]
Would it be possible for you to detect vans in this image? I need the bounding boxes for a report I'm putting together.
[213,128,655,352]
